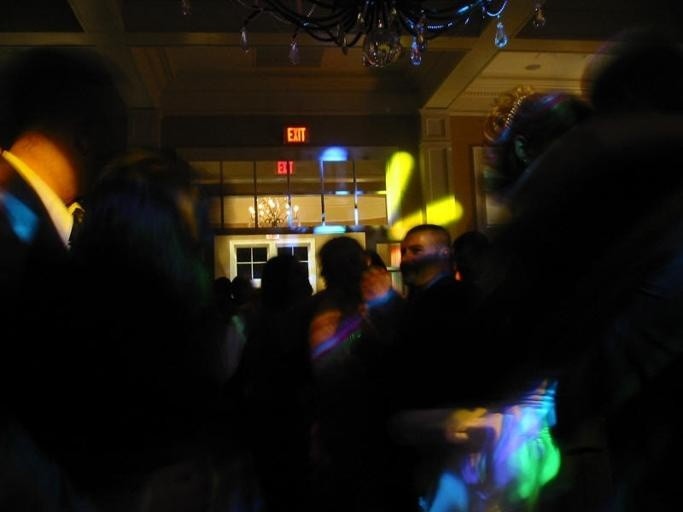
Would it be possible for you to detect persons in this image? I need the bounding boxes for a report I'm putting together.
[2,31,683,511]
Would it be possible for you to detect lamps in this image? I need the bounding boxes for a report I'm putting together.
[181,0,546,66]
